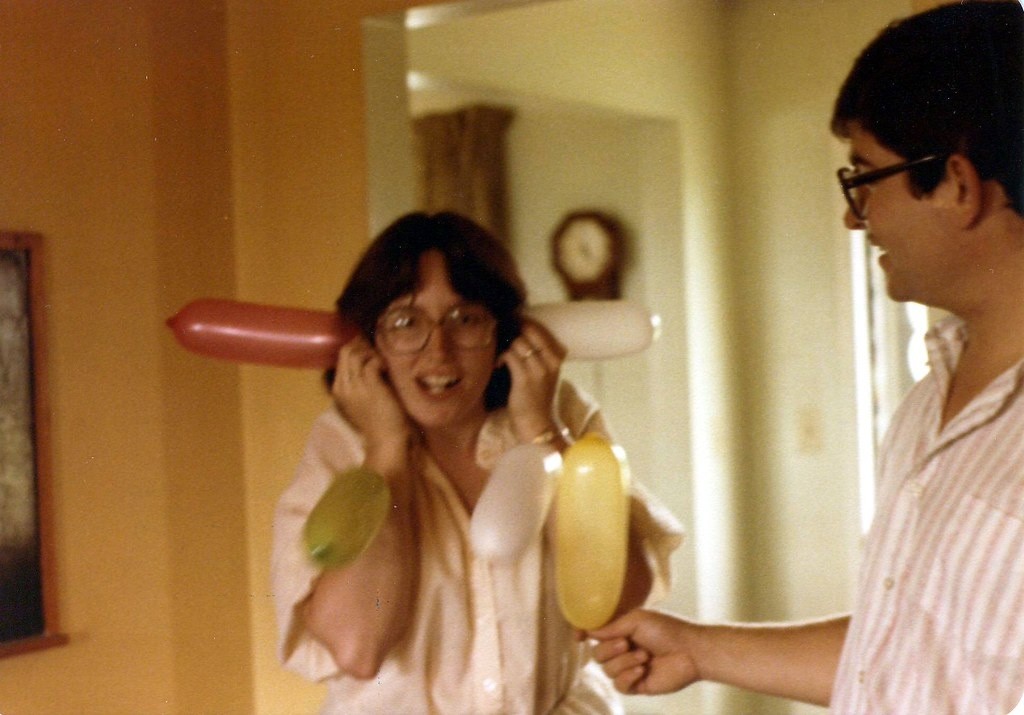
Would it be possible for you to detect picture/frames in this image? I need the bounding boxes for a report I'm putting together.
[0,232,69,658]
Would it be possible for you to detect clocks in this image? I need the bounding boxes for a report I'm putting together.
[551,210,625,303]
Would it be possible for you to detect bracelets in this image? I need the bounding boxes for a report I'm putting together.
[533,425,571,443]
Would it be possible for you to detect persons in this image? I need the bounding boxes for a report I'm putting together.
[574,2,1024,715]
[266,212,689,715]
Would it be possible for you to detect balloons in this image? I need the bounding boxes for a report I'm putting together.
[529,300,662,360]
[557,432,630,632]
[162,300,365,369]
[467,438,567,559]
[302,465,392,570]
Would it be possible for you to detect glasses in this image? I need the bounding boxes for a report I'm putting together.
[372,305,499,353]
[835,151,989,219]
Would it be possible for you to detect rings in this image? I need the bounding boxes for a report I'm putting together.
[520,347,538,361]
